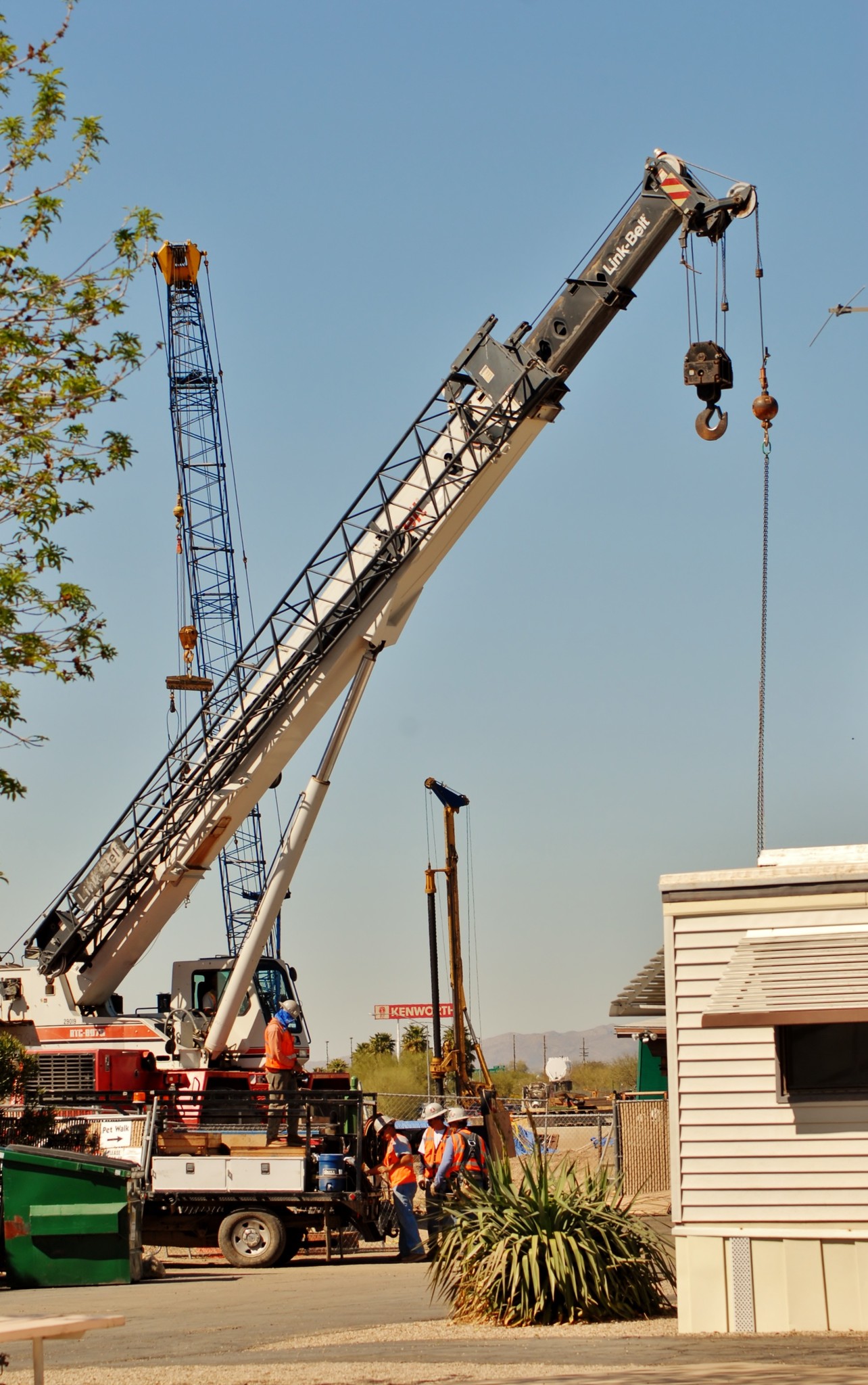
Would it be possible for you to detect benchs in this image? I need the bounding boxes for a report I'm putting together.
[0,1314,126,1385]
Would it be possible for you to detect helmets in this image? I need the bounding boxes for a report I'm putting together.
[374,1115,396,1136]
[279,999,300,1021]
[421,1102,448,1120]
[446,1108,470,1122]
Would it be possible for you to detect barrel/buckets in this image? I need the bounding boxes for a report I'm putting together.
[318,1153,343,1191]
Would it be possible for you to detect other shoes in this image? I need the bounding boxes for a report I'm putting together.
[401,1253,427,1263]
[265,1140,288,1147]
[288,1140,306,1147]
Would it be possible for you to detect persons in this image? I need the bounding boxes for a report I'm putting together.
[417,1103,454,1256]
[431,1107,490,1199]
[264,1000,310,1147]
[366,1115,427,1263]
[203,975,217,1016]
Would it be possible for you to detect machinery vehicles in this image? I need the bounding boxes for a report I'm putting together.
[99,232,364,1119]
[521,1080,599,1116]
[1,148,783,1264]
[424,776,516,1173]
